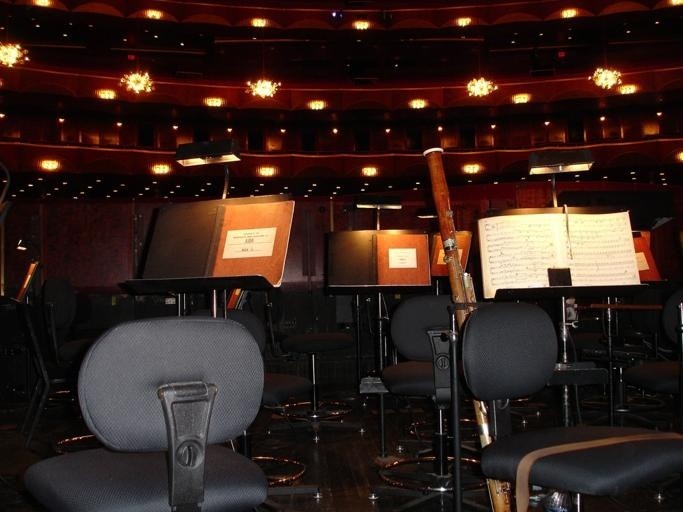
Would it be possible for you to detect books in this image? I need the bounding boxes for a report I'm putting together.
[430,230,472,277]
[477,212,641,299]
[372,234,431,285]
[203,200,295,288]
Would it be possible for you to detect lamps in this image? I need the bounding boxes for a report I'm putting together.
[241,17,282,101]
[464,8,498,97]
[118,9,156,95]
[0,0,31,68]
[591,6,622,89]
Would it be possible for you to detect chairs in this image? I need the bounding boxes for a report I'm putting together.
[39,276,97,365]
[245,297,353,419]
[20,315,278,512]
[622,291,682,428]
[195,309,311,487]
[460,300,682,512]
[0,296,78,444]
[378,292,485,492]
